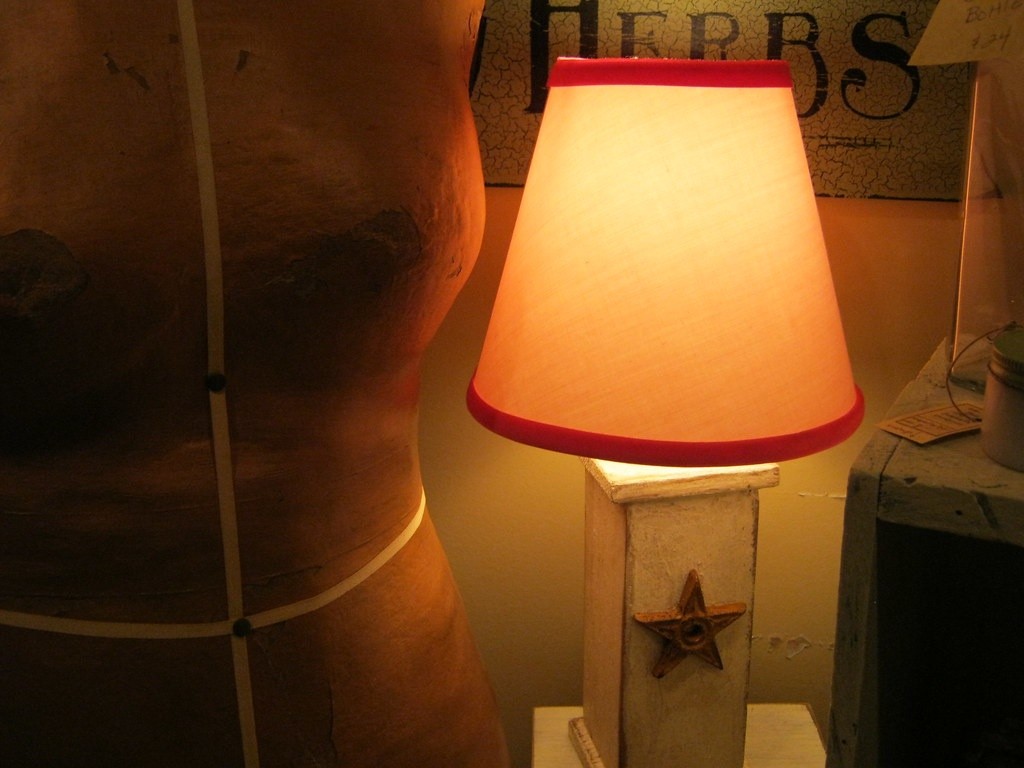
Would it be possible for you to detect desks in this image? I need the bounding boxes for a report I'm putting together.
[832,334,1024,768]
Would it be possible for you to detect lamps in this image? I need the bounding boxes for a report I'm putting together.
[467,55,869,768]
